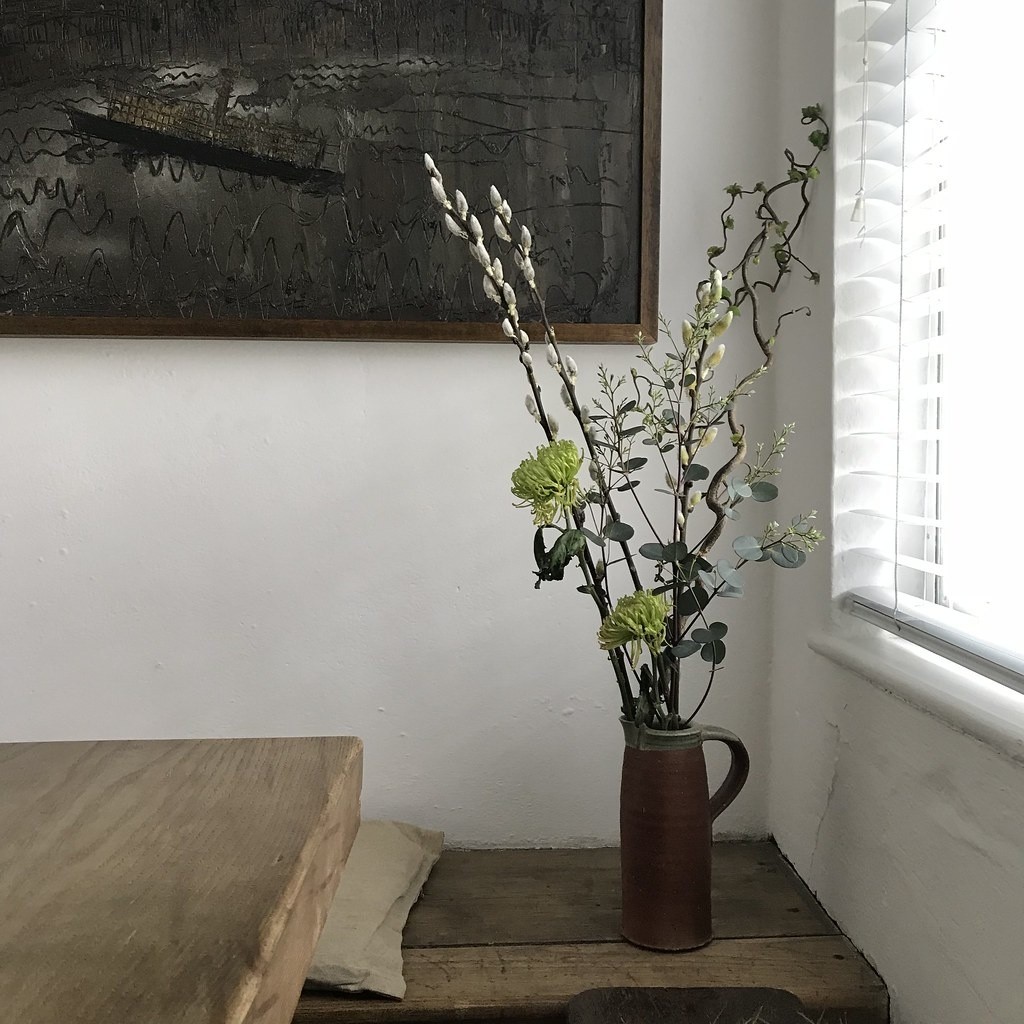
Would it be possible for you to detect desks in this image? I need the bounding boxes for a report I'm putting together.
[0,735,366,1024]
[296,839,890,1024]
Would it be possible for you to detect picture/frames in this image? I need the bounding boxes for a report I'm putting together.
[0,0,664,346]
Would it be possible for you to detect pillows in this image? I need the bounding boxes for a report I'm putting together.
[305,820,444,999]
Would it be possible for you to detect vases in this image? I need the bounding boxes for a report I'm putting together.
[618,713,747,953]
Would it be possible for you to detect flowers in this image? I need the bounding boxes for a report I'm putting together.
[421,106,832,722]
